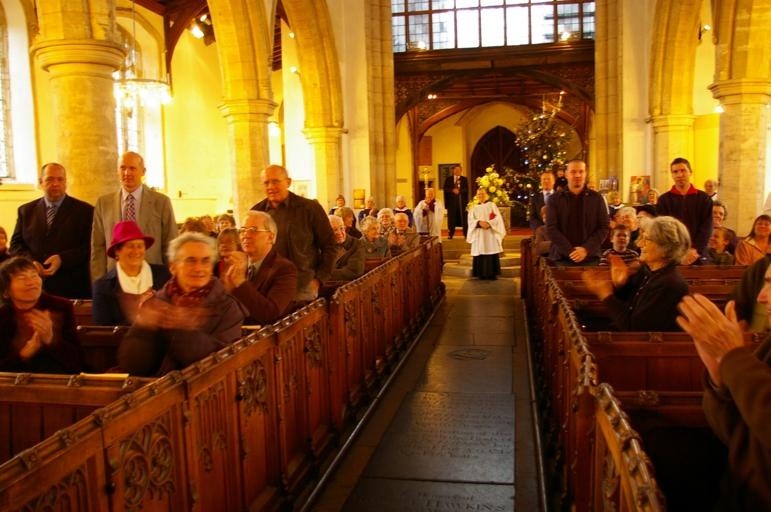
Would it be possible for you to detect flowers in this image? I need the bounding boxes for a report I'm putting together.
[465,164,514,212]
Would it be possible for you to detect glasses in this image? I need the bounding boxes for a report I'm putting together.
[640,236,653,242]
[240,227,270,233]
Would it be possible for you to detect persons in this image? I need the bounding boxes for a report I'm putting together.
[8,161,96,298]
[674,260,771,512]
[91,149,444,379]
[0,226,10,261]
[442,162,470,240]
[465,187,507,280]
[528,155,770,330]
[0,255,84,376]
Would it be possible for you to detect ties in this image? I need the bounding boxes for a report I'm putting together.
[48,205,56,226]
[125,194,135,220]
[247,266,256,282]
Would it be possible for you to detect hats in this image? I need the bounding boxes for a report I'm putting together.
[632,203,657,217]
[106,221,154,258]
